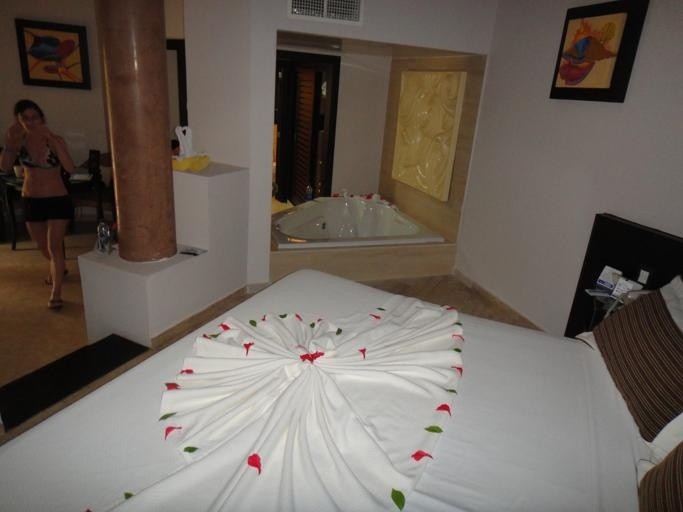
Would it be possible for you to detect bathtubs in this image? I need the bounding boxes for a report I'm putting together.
[271,194,446,250]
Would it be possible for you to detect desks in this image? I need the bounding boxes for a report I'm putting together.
[0,174,105,259]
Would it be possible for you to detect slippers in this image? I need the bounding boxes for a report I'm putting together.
[48,299,63,311]
[45,269,70,285]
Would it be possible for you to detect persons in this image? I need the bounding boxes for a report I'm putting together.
[0,99,76,308]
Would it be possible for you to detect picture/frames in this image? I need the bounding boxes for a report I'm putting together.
[13,17,90,90]
[550,1,651,103]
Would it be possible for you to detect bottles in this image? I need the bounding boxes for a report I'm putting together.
[97,220,111,254]
[305,183,312,201]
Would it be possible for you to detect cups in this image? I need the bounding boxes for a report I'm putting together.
[12,165,23,178]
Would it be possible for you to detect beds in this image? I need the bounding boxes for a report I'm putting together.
[1,268,683,510]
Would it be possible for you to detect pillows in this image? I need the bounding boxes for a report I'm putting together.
[571,273,681,511]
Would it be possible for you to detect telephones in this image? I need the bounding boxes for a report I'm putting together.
[622,290,653,307]
